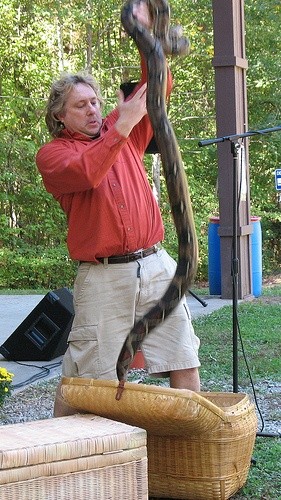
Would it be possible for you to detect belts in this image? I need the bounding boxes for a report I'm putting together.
[80,242,163,265]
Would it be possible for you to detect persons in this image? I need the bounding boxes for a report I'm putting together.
[35,0,203,419]
